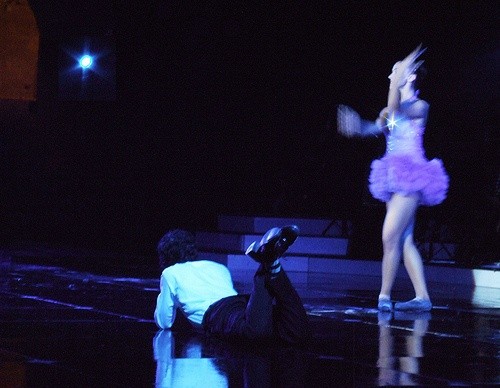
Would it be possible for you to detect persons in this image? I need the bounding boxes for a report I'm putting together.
[153,225,315,348]
[335,46,449,312]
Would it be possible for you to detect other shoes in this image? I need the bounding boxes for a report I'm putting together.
[378,295,392,312]
[245,225,300,264]
[394,298,433,312]
